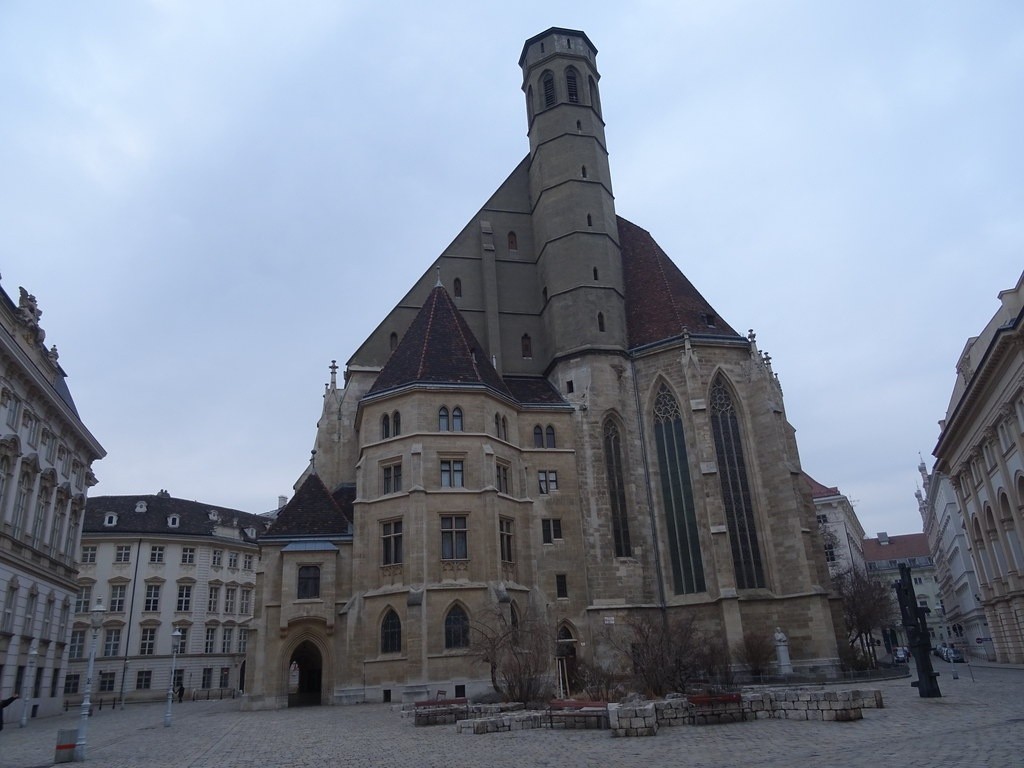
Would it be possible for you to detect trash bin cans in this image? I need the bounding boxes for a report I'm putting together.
[53,728,79,764]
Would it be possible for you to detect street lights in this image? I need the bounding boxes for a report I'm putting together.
[71,596,107,763]
[162,624,183,726]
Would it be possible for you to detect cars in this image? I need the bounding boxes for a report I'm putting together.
[933,644,965,663]
[892,646,911,663]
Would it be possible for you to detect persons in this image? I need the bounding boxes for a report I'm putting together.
[172,684,185,702]
[0,694,18,730]
